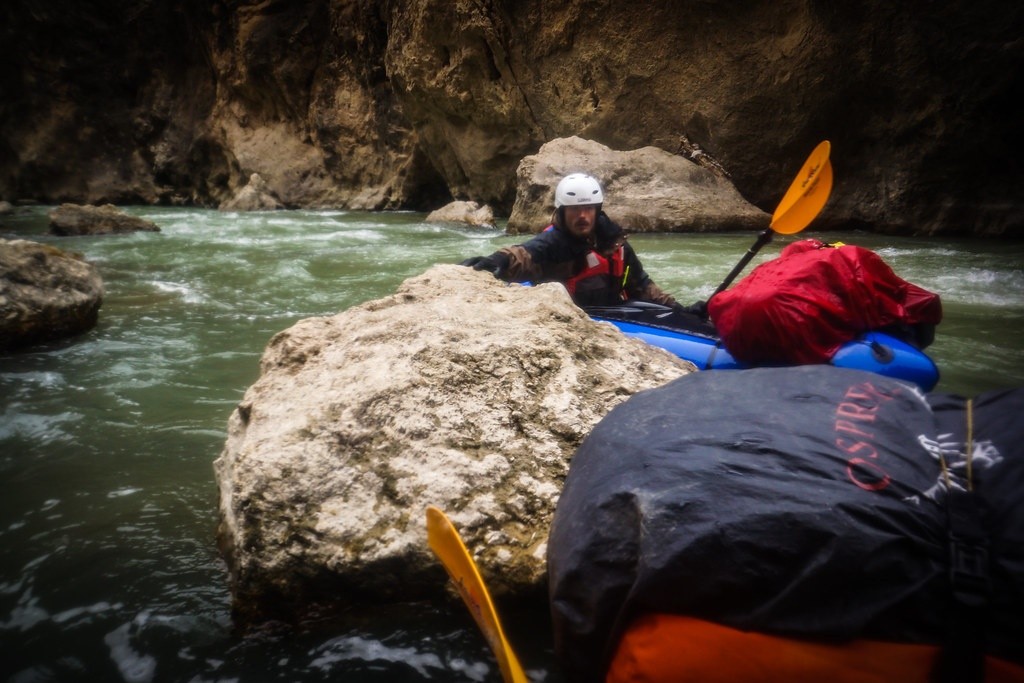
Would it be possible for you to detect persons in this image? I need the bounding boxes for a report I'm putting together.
[460,172,708,326]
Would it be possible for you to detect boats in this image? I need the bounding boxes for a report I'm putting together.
[520,276,942,393]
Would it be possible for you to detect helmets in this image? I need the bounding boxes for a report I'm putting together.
[555,173,604,209]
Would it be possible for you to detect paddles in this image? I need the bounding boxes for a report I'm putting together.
[704,136,834,307]
[425,505,529,683]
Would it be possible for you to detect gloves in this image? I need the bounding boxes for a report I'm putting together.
[458,251,509,279]
[680,301,709,321]
